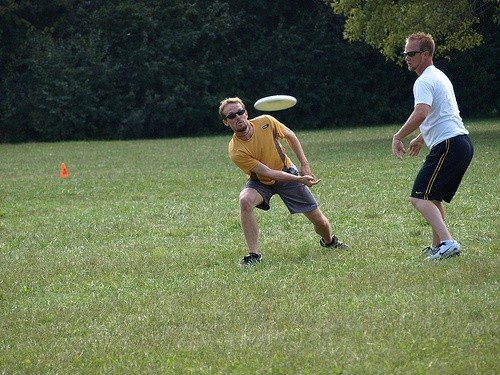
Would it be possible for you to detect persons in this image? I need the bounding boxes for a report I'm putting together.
[218,97,348,265]
[392,31,474,259]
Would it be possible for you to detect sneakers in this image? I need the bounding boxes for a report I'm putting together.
[241,253,262,264]
[424,241,460,261]
[320,236,348,249]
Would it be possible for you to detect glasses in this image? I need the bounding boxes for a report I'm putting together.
[223,108,246,119]
[403,51,426,57]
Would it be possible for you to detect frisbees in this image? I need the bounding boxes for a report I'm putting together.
[253,95,297,111]
